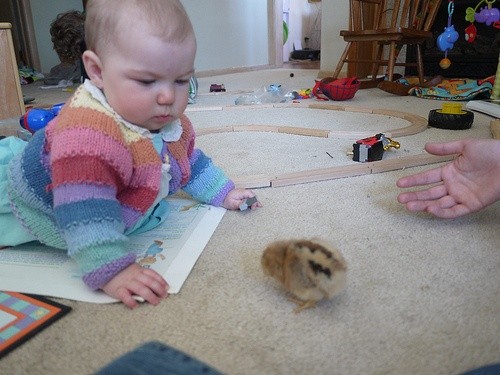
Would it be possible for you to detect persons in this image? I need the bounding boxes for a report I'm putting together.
[0,0,262,307]
[396,138,500,217]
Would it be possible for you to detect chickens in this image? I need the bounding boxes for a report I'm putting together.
[259,239,350,313]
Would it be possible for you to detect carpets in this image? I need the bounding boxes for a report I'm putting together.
[0,66,500,375]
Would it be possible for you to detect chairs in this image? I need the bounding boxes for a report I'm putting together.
[332,0,440,82]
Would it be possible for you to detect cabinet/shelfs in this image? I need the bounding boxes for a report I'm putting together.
[0,22,24,122]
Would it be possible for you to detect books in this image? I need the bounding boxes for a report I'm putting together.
[0,196,226,303]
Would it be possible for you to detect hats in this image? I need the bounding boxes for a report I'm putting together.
[313,75,360,100]
